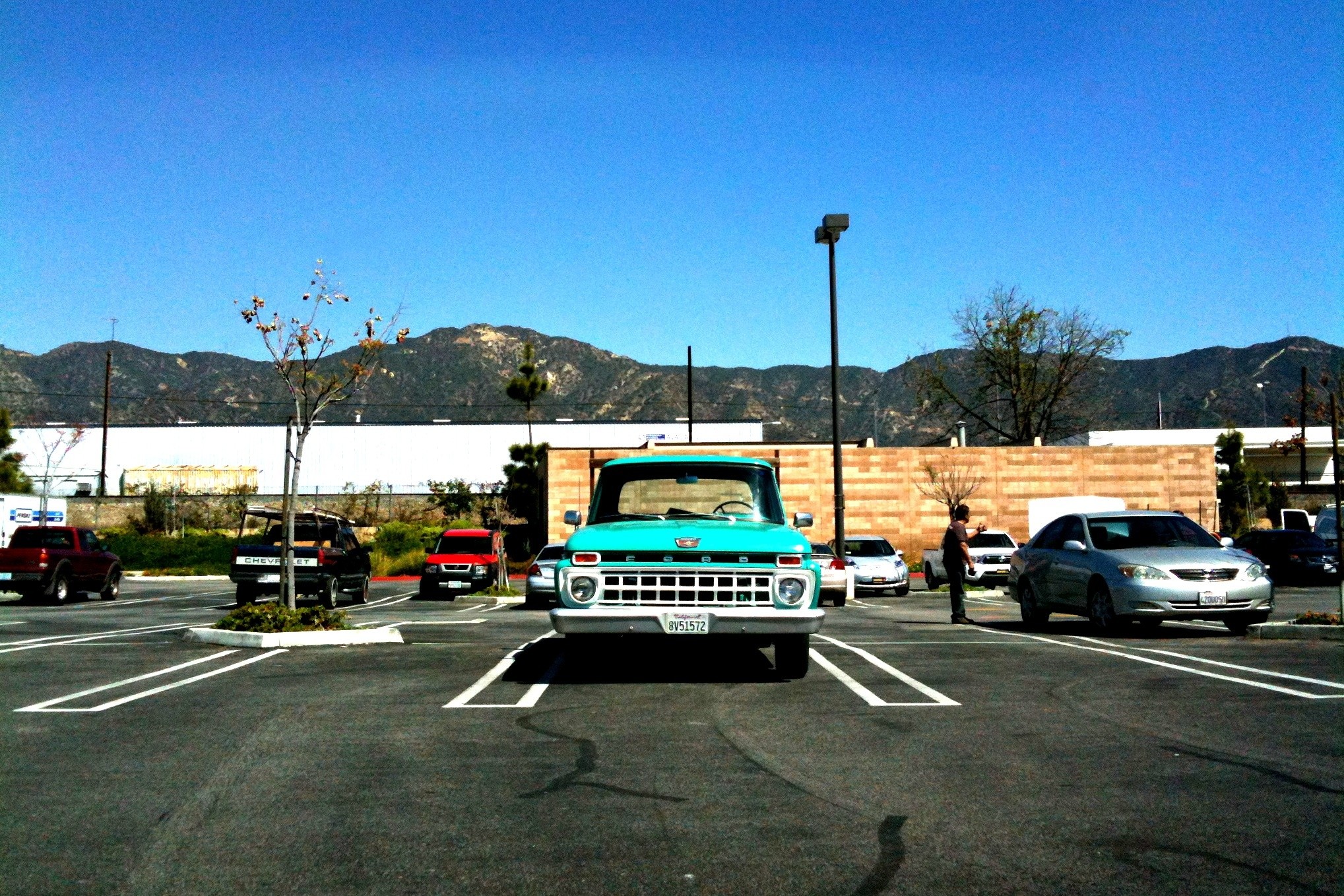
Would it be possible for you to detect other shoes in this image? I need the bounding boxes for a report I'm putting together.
[951,615,974,625]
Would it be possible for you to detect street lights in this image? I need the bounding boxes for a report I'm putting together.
[813,213,851,562]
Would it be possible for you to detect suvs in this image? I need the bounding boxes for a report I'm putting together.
[419,529,507,601]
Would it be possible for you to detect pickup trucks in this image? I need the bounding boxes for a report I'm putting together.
[229,505,374,611]
[0,526,121,606]
[548,451,826,679]
[922,529,1026,592]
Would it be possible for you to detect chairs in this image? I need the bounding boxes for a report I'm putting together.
[1147,520,1172,547]
[1079,527,1110,547]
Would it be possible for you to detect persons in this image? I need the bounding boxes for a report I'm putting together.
[941,505,987,624]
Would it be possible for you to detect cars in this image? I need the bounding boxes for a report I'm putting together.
[827,538,909,597]
[1008,510,1274,640]
[809,544,848,606]
[1314,503,1344,540]
[526,544,565,609]
[1233,528,1340,587]
